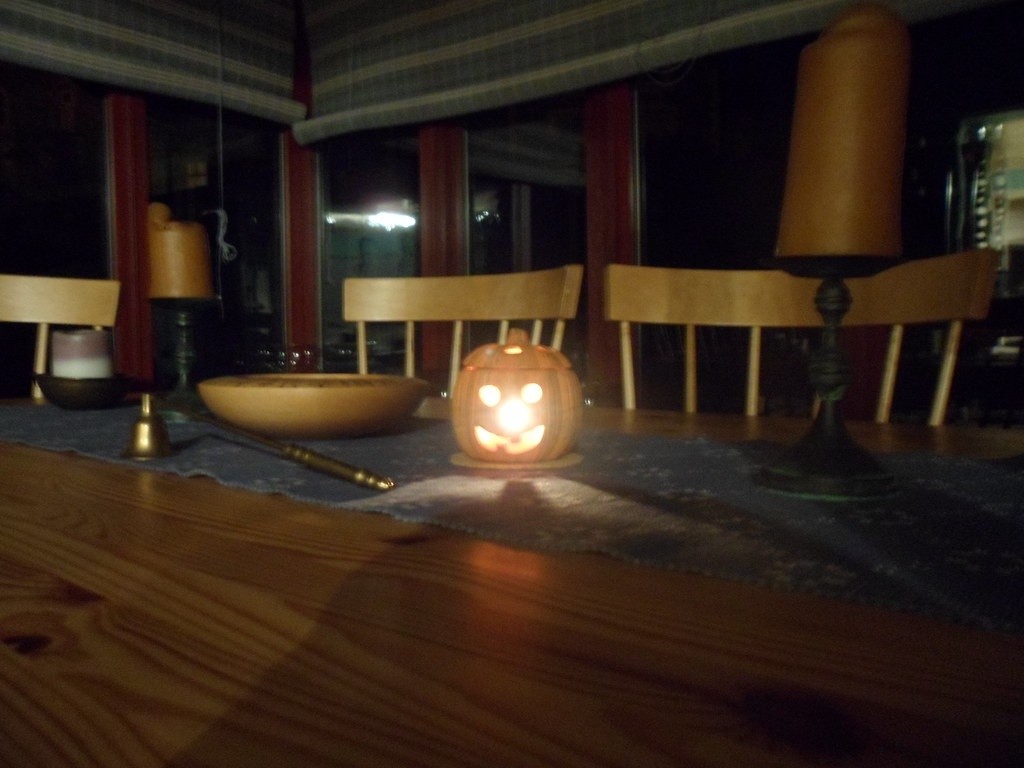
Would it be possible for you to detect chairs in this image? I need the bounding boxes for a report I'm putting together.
[345,263,584,394]
[1,276,122,400]
[599,247,1002,427]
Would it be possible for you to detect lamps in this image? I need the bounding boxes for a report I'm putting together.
[745,1,912,505]
[450,328,586,465]
[147,221,217,400]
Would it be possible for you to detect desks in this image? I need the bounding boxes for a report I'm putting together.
[3,394,1024,762]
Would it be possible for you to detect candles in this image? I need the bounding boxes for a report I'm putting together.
[51,330,117,380]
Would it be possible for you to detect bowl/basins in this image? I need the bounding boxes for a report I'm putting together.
[198,373,430,439]
